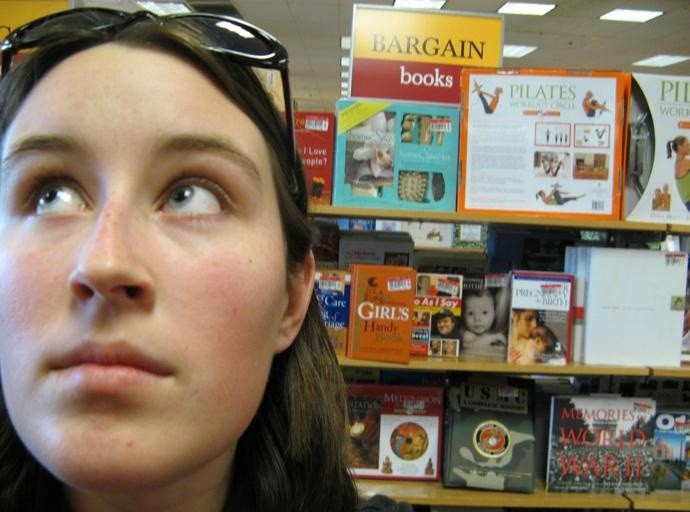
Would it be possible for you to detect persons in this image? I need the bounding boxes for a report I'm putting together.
[0,7,442,510]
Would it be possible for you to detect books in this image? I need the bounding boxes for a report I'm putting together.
[312,230,690,495]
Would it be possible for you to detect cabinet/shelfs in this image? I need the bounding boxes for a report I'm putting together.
[298,200,690,512]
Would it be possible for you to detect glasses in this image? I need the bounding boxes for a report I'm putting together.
[1,8,297,170]
